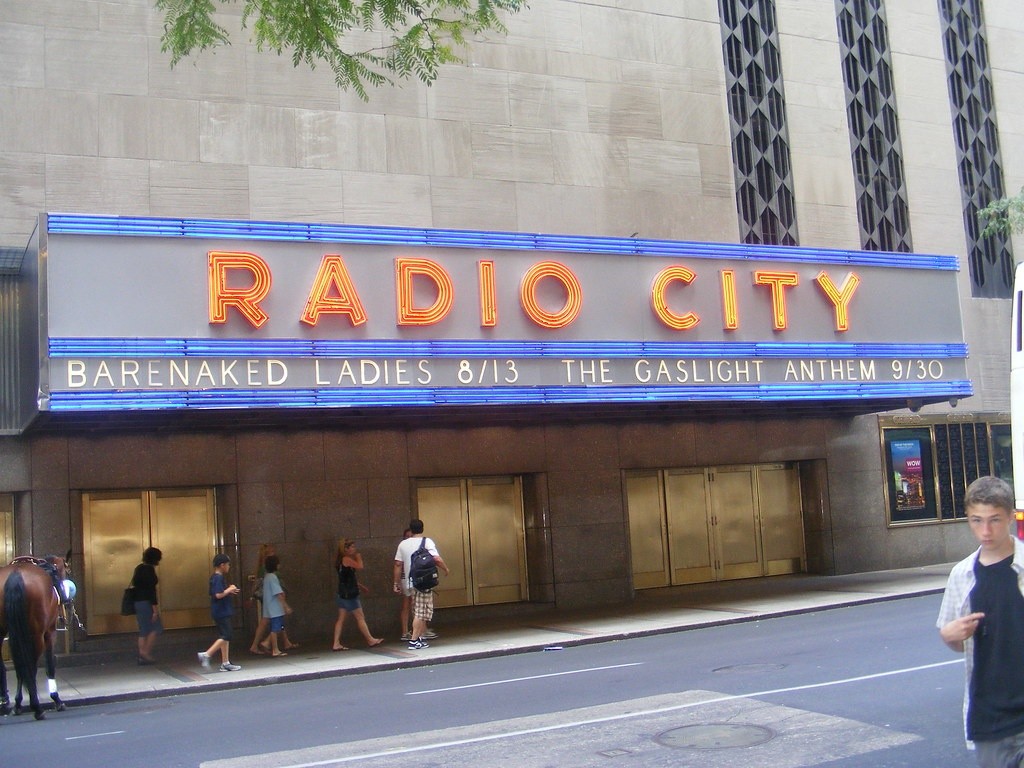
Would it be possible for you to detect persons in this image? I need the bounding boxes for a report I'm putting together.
[250,555,301,657]
[196,554,242,673]
[936,476,1023,768]
[131,546,165,667]
[393,518,449,650]
[332,536,385,651]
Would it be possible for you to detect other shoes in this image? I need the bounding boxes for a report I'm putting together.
[138,654,162,665]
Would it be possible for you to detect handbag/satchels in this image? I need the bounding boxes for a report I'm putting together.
[121,578,137,616]
[254,573,288,600]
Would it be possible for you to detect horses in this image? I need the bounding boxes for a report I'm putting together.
[0,549,73,720]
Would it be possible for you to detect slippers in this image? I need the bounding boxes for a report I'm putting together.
[248,648,266,656]
[260,641,272,654]
[333,646,350,652]
[368,639,384,647]
[271,651,289,658]
[282,643,300,651]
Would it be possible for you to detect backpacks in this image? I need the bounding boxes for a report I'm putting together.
[409,537,439,595]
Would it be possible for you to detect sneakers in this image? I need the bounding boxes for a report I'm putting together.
[401,631,412,641]
[197,652,213,673]
[220,658,242,672]
[422,632,439,640]
[408,637,429,649]
